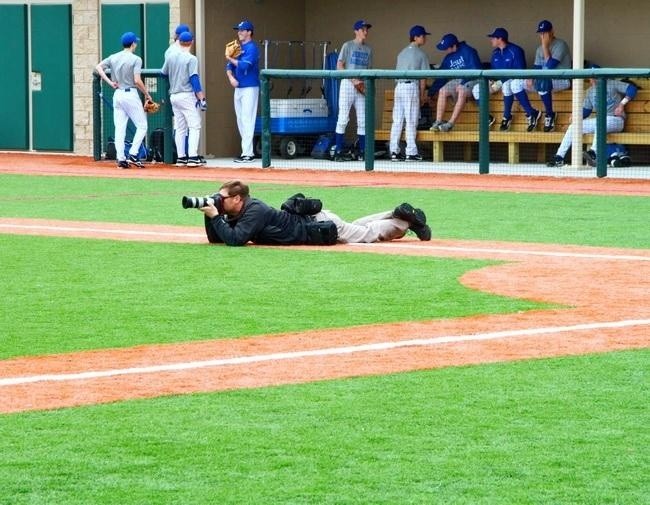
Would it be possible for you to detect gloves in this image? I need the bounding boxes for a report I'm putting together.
[195,98,207,111]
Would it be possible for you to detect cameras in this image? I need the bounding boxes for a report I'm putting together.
[182,193,223,215]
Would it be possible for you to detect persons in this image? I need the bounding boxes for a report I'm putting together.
[226,23,261,163]
[427,31,484,132]
[334,20,373,161]
[95,31,153,168]
[161,31,207,167]
[544,64,637,168]
[197,179,433,247]
[471,28,526,131]
[510,20,572,132]
[169,24,204,161]
[387,25,431,162]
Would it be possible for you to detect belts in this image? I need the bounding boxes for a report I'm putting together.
[125,88,130,92]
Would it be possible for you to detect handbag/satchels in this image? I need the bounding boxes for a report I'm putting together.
[417,103,432,130]
[106,137,148,161]
[306,219,337,245]
[280,193,323,216]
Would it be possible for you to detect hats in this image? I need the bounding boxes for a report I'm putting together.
[121,32,141,45]
[233,20,253,31]
[410,25,431,36]
[488,28,508,40]
[354,20,372,30]
[436,34,457,50]
[536,20,552,32]
[176,24,193,42]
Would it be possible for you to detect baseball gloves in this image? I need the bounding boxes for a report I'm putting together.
[143,99,160,112]
[225,40,242,58]
[355,80,365,95]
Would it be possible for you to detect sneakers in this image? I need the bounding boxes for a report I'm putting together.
[392,203,426,229]
[500,114,513,130]
[406,153,423,162]
[176,158,188,167]
[489,115,494,128]
[409,221,431,241]
[525,110,541,131]
[234,156,256,163]
[337,150,353,160]
[547,155,565,168]
[583,149,596,167]
[430,120,453,131]
[391,151,405,162]
[118,161,132,169]
[187,155,206,167]
[128,154,145,168]
[545,112,557,132]
[358,153,365,161]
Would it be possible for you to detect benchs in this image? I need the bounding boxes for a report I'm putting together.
[374,78,650,162]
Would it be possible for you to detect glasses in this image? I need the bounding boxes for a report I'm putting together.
[222,194,242,204]
